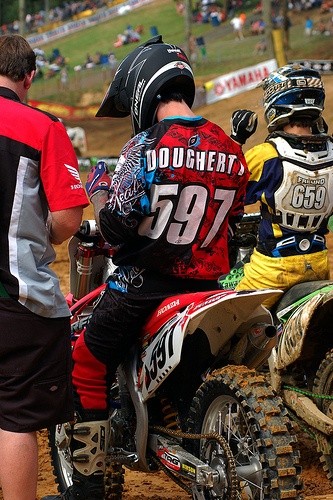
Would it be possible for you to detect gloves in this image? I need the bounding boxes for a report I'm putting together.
[311,116,328,134]
[230,109,258,145]
[85,161,111,204]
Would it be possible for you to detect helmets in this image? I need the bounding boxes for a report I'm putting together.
[257,64,325,133]
[95,35,196,136]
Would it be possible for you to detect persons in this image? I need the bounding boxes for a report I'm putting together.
[41,34,250,500]
[229,64,333,310]
[0,34,91,500]
[0,0,333,89]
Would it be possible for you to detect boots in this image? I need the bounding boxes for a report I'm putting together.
[41,418,112,500]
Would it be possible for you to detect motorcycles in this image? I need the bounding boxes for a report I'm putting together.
[45,220,303,495]
[232,213,333,484]
